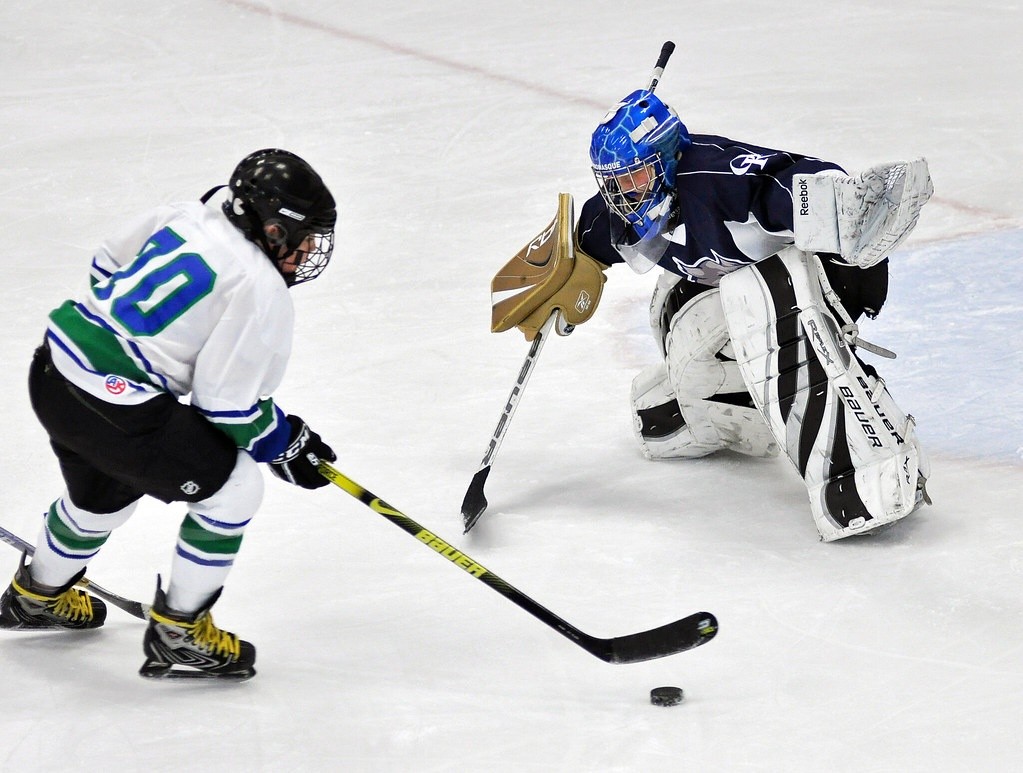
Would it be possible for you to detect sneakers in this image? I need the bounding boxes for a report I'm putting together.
[0,549,107,631]
[854,470,926,537]
[138,574,256,682]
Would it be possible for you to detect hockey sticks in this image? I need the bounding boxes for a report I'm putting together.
[0,524,162,618]
[460,40,677,536]
[314,457,718,665]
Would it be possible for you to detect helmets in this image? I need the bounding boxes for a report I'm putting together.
[589,88,690,275]
[222,148,337,288]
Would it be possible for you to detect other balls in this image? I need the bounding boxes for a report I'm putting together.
[650,686,683,708]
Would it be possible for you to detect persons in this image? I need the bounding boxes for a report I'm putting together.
[0,148,338,681]
[489,88,934,541]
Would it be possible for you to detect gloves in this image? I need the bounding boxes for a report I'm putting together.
[267,414,337,489]
